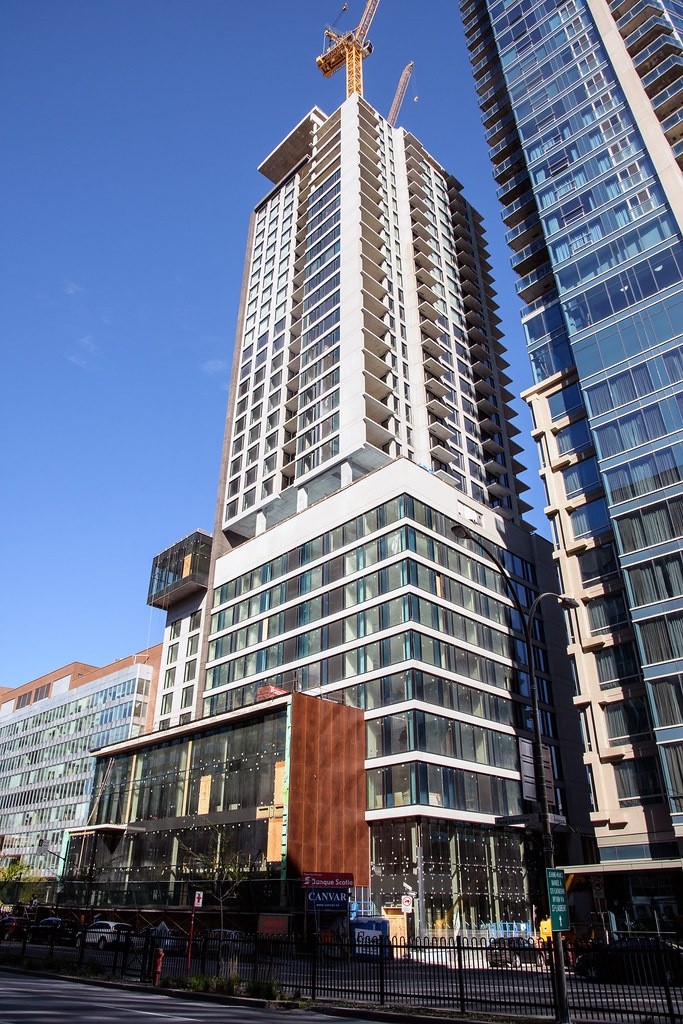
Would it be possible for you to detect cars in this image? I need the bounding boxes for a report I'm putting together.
[26,917,77,946]
[190,927,255,962]
[129,926,185,956]
[74,921,132,952]
[574,937,683,986]
[486,937,545,968]
[0,916,33,942]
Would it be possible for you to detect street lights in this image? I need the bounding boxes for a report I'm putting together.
[448,524,583,1024]
